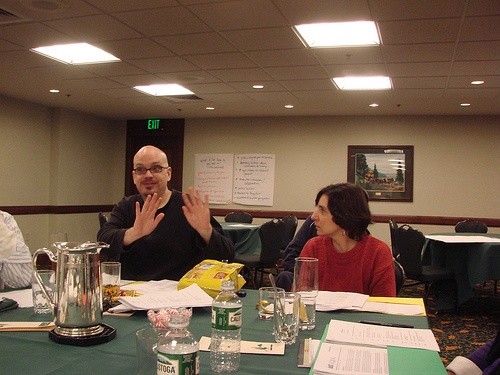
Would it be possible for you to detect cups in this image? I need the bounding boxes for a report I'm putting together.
[32,270,56,313]
[258,287,285,320]
[273,292,301,345]
[100,262,121,302]
[135,326,189,375]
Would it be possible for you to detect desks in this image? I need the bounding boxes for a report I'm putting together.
[422,233,500,307]
[0,281,447,375]
[220,221,262,275]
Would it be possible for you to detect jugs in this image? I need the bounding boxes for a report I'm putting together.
[31,242,110,338]
[292,256,319,330]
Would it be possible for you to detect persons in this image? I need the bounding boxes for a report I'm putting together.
[291,183,396,298]
[96,145,235,281]
[445,326,500,375]
[0,210,33,291]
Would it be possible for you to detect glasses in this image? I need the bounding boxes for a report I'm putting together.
[133,165,170,175]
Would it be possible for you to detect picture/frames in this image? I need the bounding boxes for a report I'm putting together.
[347,144,413,201]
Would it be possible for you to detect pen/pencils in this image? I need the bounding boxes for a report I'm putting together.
[358,320,414,328]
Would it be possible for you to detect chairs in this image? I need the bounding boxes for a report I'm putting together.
[98,211,110,262]
[393,258,406,296]
[234,219,284,288]
[454,219,487,233]
[225,211,254,224]
[389,217,398,252]
[393,224,459,311]
[274,213,298,274]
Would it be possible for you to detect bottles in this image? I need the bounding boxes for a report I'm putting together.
[210,281,243,373]
[155,315,200,375]
